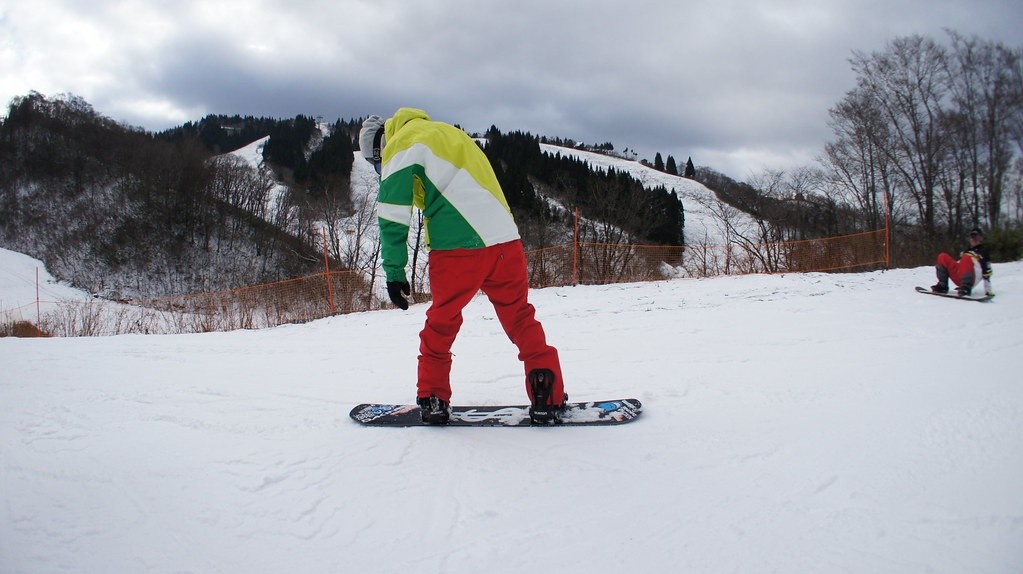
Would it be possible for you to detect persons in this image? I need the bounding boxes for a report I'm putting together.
[931,227,994,296]
[359,107,571,421]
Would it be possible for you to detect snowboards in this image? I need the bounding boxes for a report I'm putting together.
[915,286,994,301]
[349,398,641,427]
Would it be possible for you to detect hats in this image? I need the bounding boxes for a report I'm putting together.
[971,228,982,236]
[359,116,388,164]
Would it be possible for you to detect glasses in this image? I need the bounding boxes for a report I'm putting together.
[373,158,382,175]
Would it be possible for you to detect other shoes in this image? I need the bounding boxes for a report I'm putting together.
[931,282,948,293]
[417,396,449,423]
[956,286,970,296]
[529,369,568,426]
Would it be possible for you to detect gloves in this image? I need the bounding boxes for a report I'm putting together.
[388,280,411,310]
[985,291,995,298]
